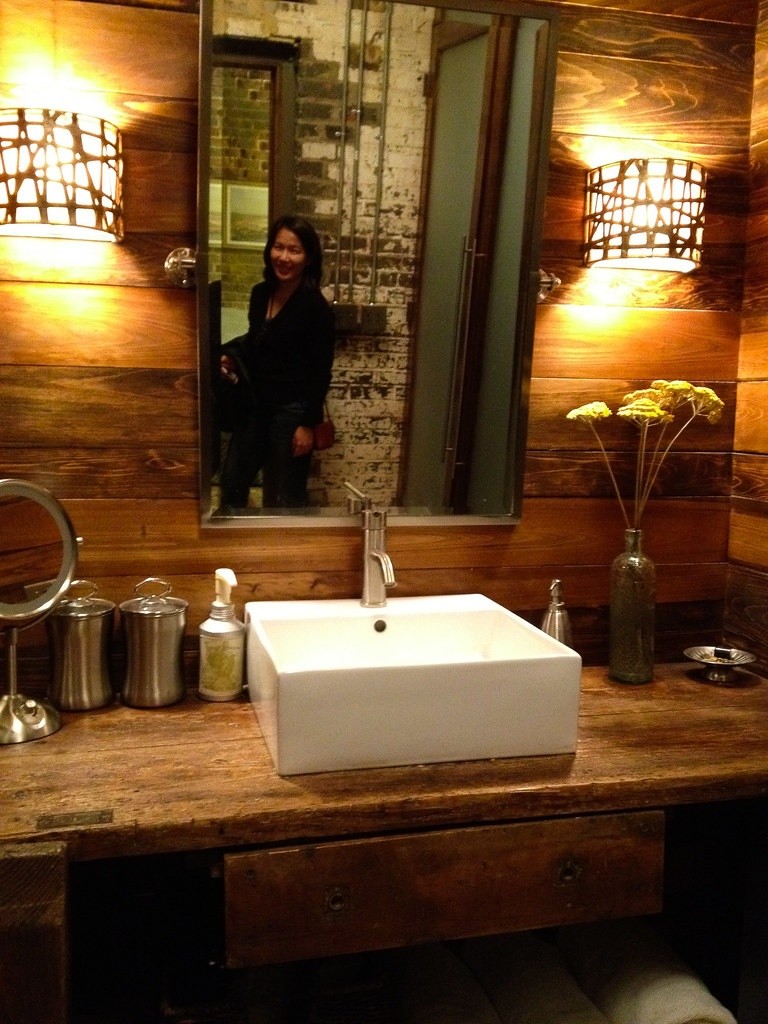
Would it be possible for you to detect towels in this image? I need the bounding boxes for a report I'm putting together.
[408,926,740,1022]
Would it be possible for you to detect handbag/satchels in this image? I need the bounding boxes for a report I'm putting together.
[313,419,336,450]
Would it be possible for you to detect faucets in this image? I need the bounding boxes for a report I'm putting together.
[342,481,374,519]
[364,506,395,604]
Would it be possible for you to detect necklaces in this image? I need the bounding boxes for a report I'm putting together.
[269,293,276,318]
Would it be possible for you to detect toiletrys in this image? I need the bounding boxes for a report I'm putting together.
[199,568,250,702]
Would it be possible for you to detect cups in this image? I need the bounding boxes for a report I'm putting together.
[52,599,115,710]
[120,598,187,707]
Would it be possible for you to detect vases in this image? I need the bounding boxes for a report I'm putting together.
[609,528,656,684]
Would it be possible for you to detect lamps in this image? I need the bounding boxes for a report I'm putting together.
[582,158,709,272]
[1,106,125,244]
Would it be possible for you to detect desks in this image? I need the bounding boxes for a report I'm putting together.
[0,655,768,1024]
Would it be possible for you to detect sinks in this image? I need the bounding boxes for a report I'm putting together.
[245,592,582,777]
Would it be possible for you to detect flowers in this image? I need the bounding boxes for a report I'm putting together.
[565,379,727,529]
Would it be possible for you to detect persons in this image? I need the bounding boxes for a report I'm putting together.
[217,212,336,513]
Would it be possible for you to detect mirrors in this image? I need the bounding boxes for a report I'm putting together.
[195,0,561,531]
